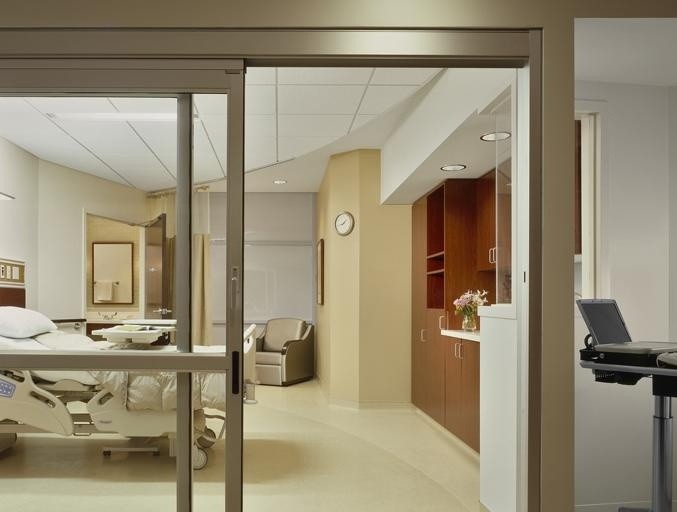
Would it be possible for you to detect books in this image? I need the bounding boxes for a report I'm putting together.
[108,325,166,331]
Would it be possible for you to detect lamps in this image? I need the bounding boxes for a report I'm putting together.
[439,164,466,171]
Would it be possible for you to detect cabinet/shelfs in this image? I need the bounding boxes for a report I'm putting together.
[410,178,442,435]
[443,336,479,455]
[477,157,510,271]
[477,303,517,512]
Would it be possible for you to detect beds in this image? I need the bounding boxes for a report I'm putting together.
[0,305,258,470]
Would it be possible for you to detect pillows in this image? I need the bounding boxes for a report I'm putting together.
[0,305,57,340]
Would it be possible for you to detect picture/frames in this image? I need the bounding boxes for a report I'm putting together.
[316,238,324,305]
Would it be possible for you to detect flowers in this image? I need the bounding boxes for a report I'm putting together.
[452,288,488,315]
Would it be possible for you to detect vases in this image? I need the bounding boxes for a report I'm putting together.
[461,314,476,332]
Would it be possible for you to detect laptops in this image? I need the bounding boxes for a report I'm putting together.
[576,299,677,360]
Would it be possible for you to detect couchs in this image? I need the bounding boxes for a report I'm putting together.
[255,318,314,386]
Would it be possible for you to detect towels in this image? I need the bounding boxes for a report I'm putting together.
[96,280,112,301]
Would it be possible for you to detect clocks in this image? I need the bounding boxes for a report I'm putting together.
[334,212,354,237]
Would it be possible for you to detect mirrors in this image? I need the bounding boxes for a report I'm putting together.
[91,241,133,305]
